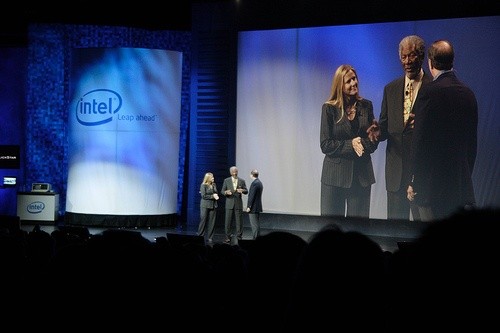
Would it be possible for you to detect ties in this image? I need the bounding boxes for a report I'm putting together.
[403,81,413,124]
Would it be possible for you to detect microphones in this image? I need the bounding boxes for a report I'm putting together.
[406,91,409,95]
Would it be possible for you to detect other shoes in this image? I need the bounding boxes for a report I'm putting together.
[209,238,213,242]
[225,238,230,242]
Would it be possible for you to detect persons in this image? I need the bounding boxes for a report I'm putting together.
[221,166,249,244]
[406,40,479,221]
[319,64,380,218]
[1,204,500,333]
[197,173,220,243]
[366,35,434,221]
[246,169,264,240]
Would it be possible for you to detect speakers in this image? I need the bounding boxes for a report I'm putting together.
[167,232,207,256]
[104,229,142,252]
[59,225,90,243]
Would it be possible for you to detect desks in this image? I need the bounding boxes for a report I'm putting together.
[16,191,59,225]
